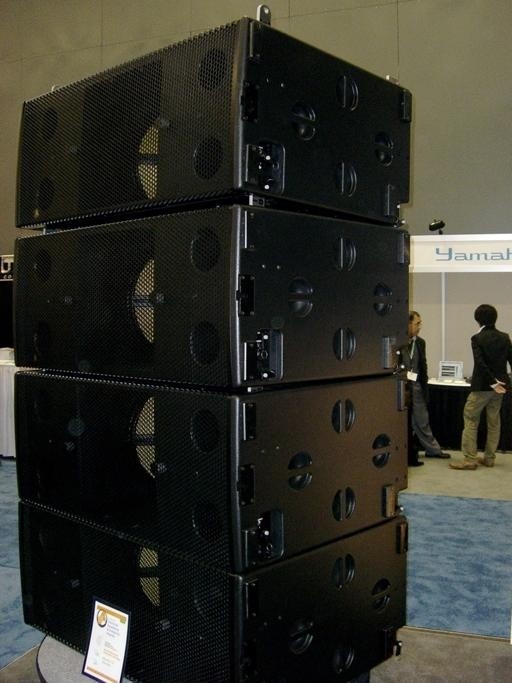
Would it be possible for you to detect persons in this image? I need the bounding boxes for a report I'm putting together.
[449,302,511,469]
[408,309,451,466]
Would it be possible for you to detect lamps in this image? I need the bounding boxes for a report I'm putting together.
[427,216,446,235]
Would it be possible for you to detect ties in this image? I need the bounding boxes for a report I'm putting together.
[408,339,414,356]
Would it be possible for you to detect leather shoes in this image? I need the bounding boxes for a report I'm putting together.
[424,451,450,459]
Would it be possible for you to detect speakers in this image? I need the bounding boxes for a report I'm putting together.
[14,18,413,682]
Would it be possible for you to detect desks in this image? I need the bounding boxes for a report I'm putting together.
[425,377,511,455]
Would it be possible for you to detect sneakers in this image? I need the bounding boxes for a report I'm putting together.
[477,454,495,467]
[447,460,478,470]
[408,460,424,466]
